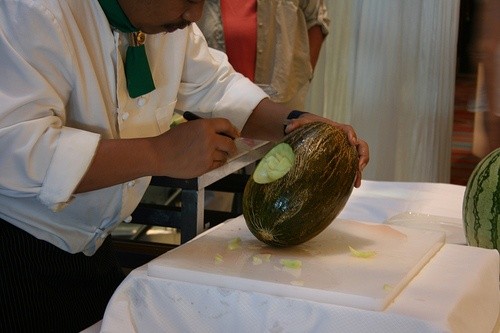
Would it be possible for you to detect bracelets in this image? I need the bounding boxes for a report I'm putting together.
[283,109,308,143]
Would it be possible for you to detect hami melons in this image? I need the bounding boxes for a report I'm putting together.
[243,122,361,248]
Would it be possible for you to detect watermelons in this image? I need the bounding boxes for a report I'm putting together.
[463,148,500,258]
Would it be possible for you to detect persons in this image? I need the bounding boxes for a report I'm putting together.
[0,0,370,333]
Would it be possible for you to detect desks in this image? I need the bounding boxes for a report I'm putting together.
[132,138,276,245]
[77,180,500,333]
